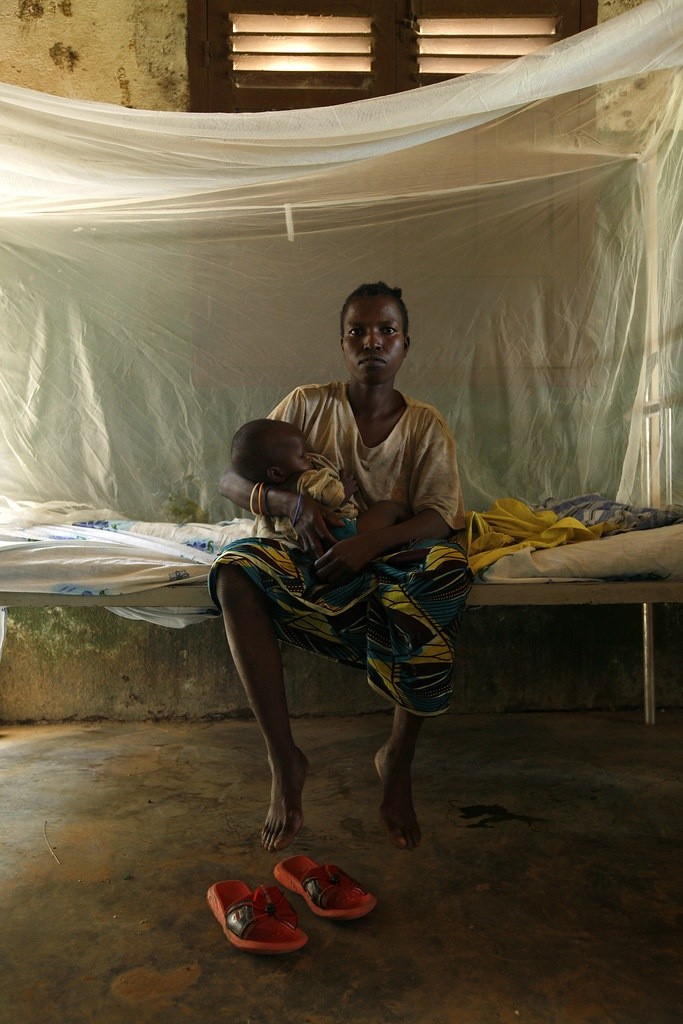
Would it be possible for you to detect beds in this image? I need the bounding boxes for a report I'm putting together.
[0,497,682,726]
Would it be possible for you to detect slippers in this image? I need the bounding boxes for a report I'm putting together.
[273,854,377,919]
[208,879,308,952]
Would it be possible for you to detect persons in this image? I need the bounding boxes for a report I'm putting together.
[208,281,474,850]
[231,419,412,555]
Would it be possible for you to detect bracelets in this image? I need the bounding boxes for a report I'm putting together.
[249,482,273,520]
[292,493,301,527]
